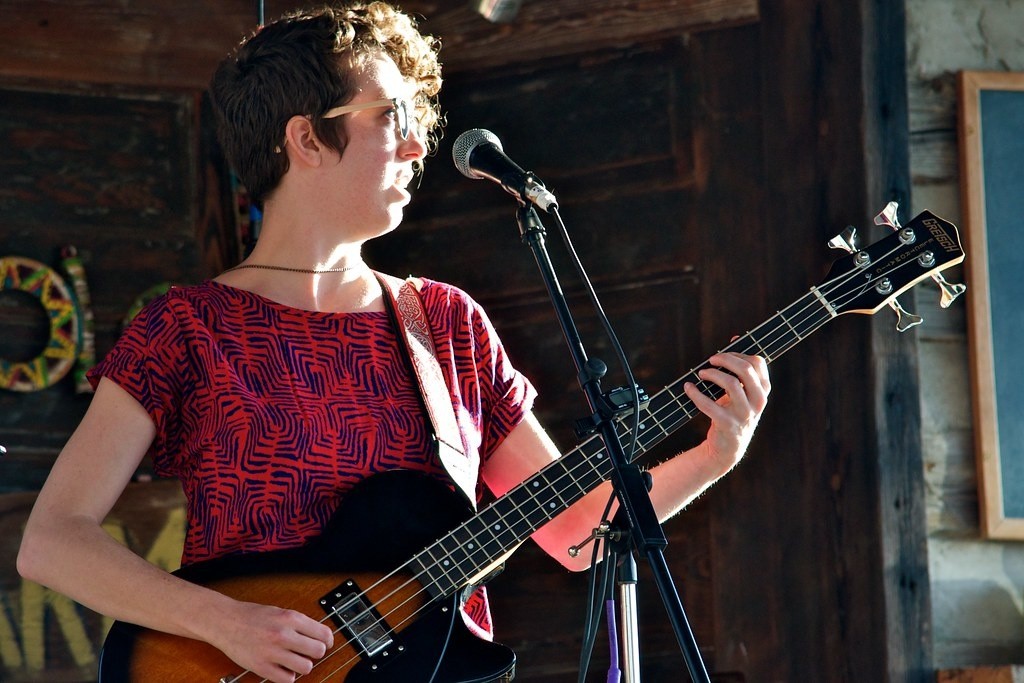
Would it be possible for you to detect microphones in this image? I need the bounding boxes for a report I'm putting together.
[451,127,560,213]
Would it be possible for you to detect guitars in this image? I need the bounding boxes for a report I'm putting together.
[96,198,972,682]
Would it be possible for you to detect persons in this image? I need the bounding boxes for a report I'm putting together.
[16,0,773,683]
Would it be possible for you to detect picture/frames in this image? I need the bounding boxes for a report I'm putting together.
[955,69,1024,544]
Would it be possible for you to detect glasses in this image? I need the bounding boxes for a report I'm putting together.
[273,98,426,154]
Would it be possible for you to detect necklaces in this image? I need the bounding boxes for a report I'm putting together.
[225,263,353,273]
